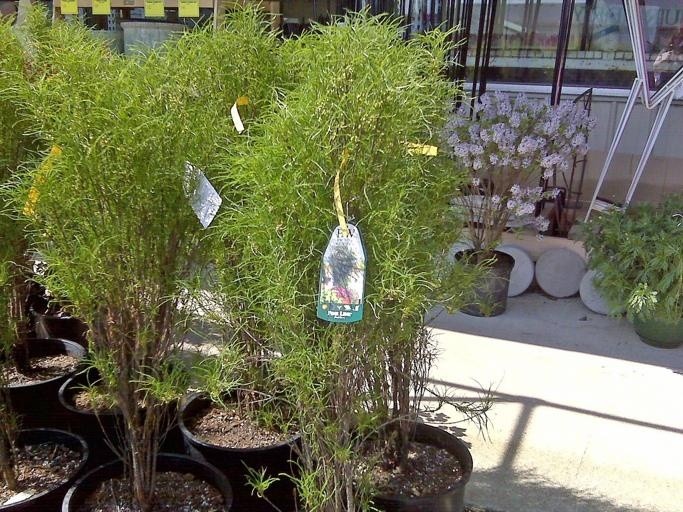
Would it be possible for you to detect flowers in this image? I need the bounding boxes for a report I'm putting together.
[445,88,598,259]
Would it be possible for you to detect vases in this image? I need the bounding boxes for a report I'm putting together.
[450,250,515,318]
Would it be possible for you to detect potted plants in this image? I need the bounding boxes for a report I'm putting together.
[0,0,475,511]
[574,183,683,351]
